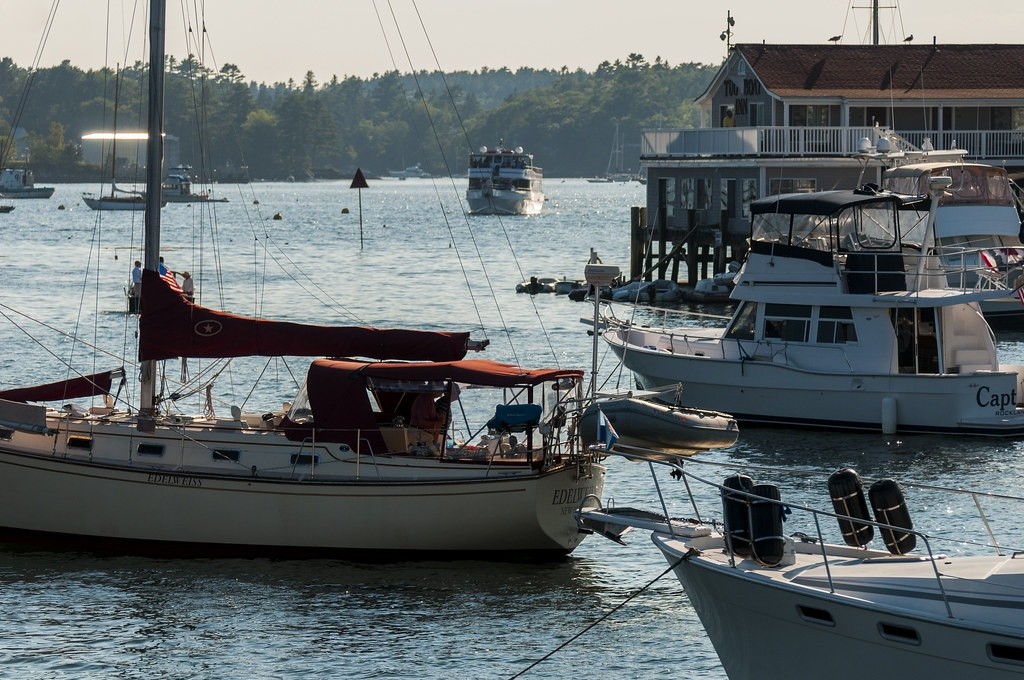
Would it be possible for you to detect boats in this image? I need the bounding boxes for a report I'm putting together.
[387,163,432,178]
[576,405,1024,680]
[579,177,1024,438]
[162,162,211,201]
[465,138,549,216]
[0,147,57,199]
[852,66,1024,320]
[580,396,739,463]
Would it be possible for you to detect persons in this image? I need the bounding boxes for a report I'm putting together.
[505,436,518,459]
[132,261,144,296]
[470,156,527,169]
[181,271,193,296]
[722,110,737,128]
[158,257,169,276]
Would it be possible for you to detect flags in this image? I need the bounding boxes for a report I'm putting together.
[595,410,619,449]
[159,262,185,296]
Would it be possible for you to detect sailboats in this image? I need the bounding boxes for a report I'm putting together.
[0,0,683,556]
[81,62,168,211]
[587,124,647,185]
